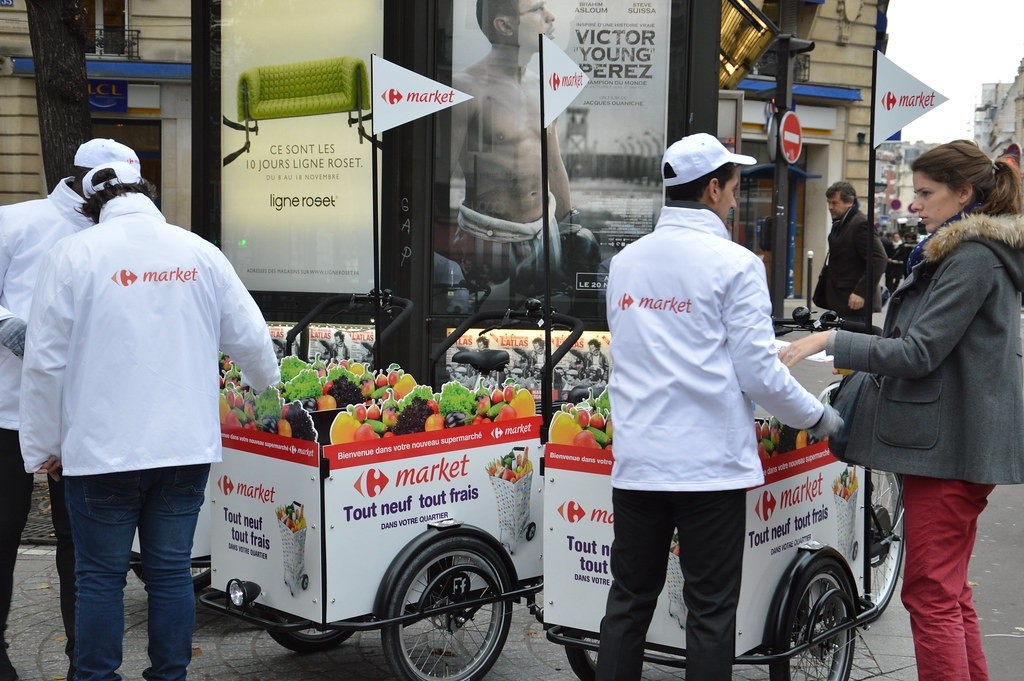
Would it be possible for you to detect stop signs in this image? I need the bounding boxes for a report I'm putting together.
[777,110,803,166]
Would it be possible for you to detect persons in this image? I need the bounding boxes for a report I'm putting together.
[879,230,919,296]
[781,141,1023,681]
[596,133,846,681]
[452,0,602,323]
[19,163,282,681]
[0,138,141,681]
[826,181,888,333]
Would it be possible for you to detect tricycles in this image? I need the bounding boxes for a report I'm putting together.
[539,306,905,681]
[117,287,586,681]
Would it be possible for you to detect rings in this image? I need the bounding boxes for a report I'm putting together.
[786,351,793,358]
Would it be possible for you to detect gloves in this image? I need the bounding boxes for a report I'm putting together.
[807,404,844,439]
[0,317,27,360]
[239,373,270,394]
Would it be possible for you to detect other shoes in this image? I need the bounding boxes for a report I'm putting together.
[114,673,122,681]
[0,646,19,681]
[142,667,159,681]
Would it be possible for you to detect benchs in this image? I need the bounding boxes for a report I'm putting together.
[236,57,370,152]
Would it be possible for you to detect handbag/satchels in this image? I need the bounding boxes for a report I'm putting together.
[812,264,836,309]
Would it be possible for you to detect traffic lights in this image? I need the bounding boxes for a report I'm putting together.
[775,34,816,110]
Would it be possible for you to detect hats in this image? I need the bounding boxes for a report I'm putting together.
[661,132,758,186]
[74,138,139,169]
[83,161,139,199]
